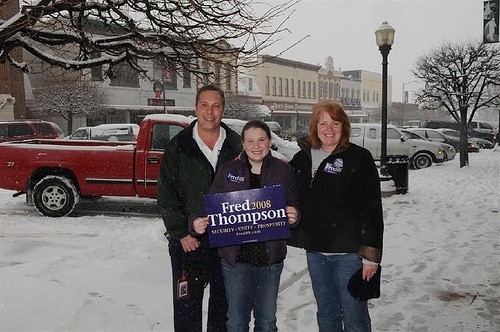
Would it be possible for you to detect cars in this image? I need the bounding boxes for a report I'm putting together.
[406,128,479,153]
[402,119,422,129]
[399,128,455,163]
[221,118,301,161]
[437,127,495,149]
[469,121,500,146]
[284,127,309,141]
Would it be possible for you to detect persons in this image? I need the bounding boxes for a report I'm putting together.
[189,120,302,332]
[155,84,242,332]
[234,102,384,332]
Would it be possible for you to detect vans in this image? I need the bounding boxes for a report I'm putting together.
[350,122,444,170]
[264,121,281,136]
[63,124,135,142]
[0,120,63,143]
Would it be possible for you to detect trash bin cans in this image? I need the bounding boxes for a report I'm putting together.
[387,153,410,194]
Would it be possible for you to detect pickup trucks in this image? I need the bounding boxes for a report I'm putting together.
[0,111,289,218]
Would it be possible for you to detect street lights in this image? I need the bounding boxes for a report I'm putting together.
[374,20,396,182]
